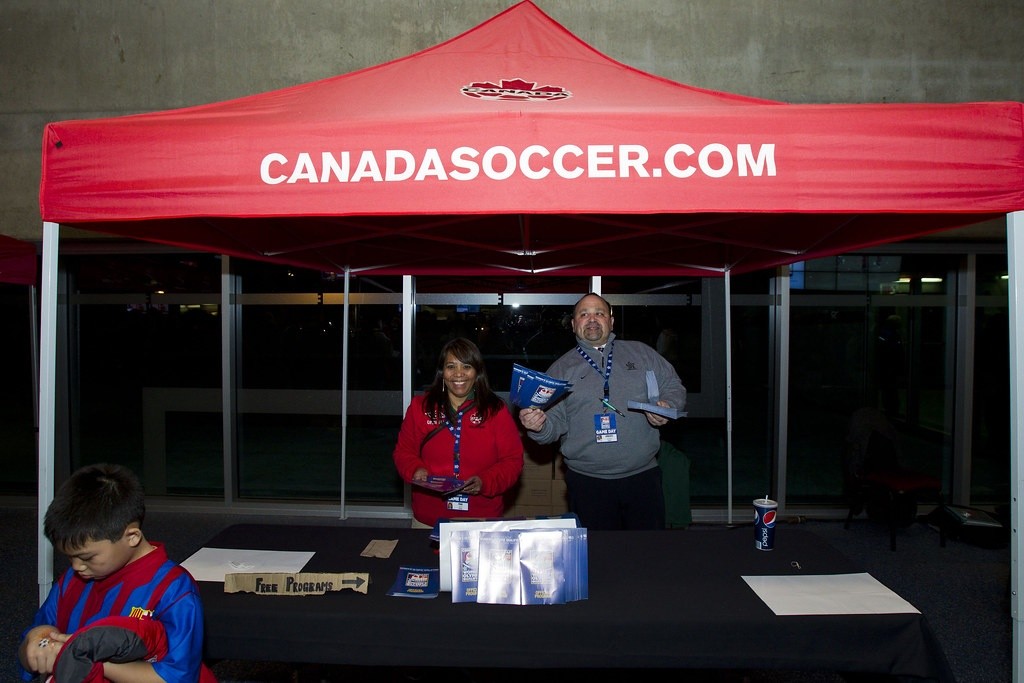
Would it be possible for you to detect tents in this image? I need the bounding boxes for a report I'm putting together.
[38,0,1024,683]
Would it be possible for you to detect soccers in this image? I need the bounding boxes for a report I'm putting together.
[39,638,51,647]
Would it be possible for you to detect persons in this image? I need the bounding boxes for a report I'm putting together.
[519,290,687,530]
[15,463,217,683]
[392,338,526,530]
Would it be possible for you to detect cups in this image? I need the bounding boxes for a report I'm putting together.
[754,499,778,550]
